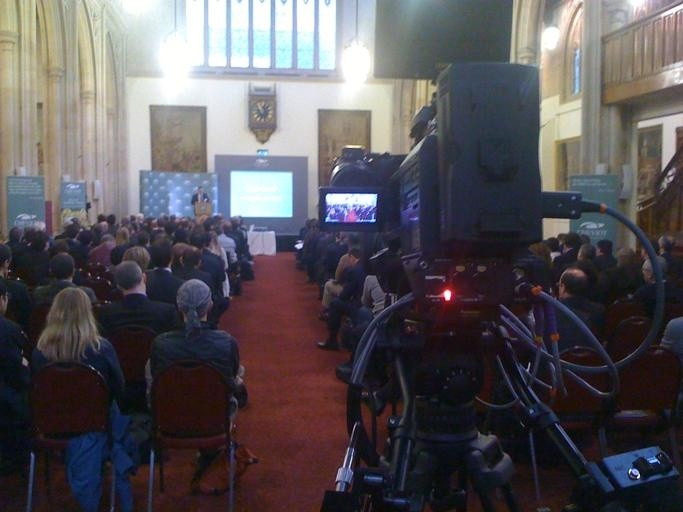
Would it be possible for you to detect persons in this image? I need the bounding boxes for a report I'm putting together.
[511,226,682,424]
[292,215,414,419]
[28,285,126,466]
[0,279,34,475]
[190,186,209,216]
[323,200,376,224]
[0,212,254,358]
[148,277,247,496]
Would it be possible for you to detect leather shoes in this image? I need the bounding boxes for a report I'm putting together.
[360,389,386,416]
[317,337,339,351]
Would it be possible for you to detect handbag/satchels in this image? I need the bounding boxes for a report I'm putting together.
[197,443,260,497]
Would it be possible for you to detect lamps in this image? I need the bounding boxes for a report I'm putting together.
[340,0,373,86]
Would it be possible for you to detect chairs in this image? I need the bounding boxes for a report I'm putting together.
[523,296,683,512]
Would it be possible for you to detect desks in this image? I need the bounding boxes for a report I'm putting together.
[246,229,276,255]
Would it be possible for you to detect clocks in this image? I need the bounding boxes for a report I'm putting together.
[249,96,277,144]
